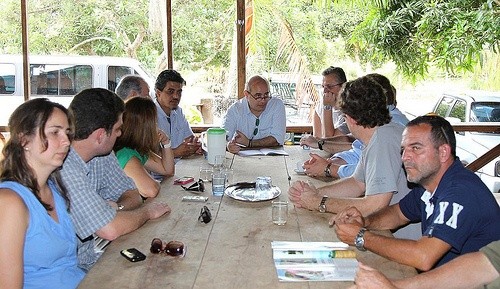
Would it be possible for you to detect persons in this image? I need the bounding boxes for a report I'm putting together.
[0,98,87,289]
[299,73,410,154]
[113,96,174,198]
[288,77,422,241]
[329,116,500,274]
[56,88,170,270]
[312,66,350,135]
[221,75,286,153]
[115,76,150,102]
[354,240,500,289]
[152,70,204,161]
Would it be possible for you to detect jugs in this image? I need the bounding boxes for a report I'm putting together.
[201,128,230,165]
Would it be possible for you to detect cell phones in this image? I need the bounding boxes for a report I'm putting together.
[120,248,146,262]
[174,176,194,185]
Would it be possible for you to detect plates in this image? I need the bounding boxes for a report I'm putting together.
[224,182,281,202]
[294,169,306,174]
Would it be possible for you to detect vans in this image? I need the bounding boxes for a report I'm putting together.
[248,72,324,112]
[0,54,204,154]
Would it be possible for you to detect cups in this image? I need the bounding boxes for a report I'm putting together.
[256,176,271,198]
[302,134,311,150]
[284,132,293,145]
[200,155,233,197]
[297,161,304,171]
[272,200,288,226]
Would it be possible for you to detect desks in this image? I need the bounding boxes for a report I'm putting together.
[76,144,418,289]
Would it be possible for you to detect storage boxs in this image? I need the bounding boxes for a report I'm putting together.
[174,177,194,185]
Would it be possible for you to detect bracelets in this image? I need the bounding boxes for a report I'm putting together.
[248,140,252,148]
[160,141,171,148]
[117,204,125,210]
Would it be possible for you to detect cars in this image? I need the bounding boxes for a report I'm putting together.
[430,89,500,194]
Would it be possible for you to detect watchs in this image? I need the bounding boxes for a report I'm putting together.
[323,105,332,110]
[319,196,327,213]
[318,140,324,150]
[355,228,368,251]
[324,162,332,177]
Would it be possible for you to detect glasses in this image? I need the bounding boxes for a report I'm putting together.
[198,205,212,223]
[247,90,272,100]
[180,177,205,193]
[321,82,343,89]
[149,237,187,258]
